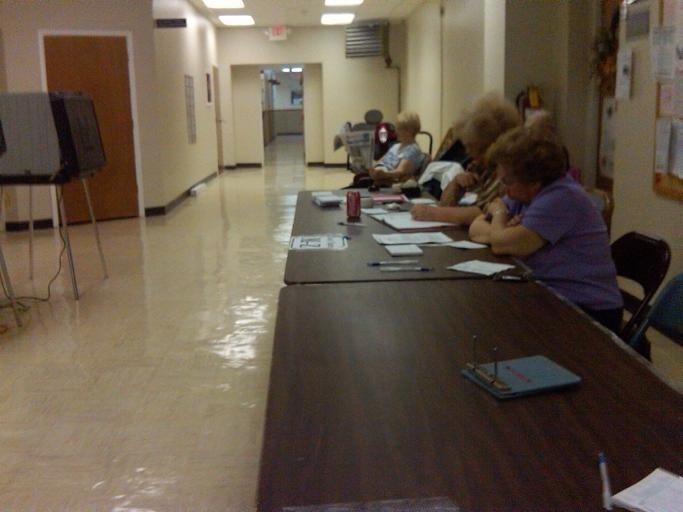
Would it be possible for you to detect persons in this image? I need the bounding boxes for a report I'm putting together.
[408,88,523,224]
[468,128,624,335]
[340,110,422,191]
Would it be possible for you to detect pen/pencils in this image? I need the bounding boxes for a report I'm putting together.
[381,267,431,271]
[367,260,419,266]
[598,452,613,511]
[337,222,366,228]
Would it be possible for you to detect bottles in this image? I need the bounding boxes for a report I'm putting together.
[347,191,361,218]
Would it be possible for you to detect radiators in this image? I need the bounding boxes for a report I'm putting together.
[345,21,389,58]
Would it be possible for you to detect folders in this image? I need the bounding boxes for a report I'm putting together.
[462,335,581,399]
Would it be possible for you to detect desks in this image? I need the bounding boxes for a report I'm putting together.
[257,280,683,512]
[284,188,536,284]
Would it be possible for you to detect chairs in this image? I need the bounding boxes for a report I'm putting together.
[630,271,683,372]
[610,225,673,364]
[584,186,614,240]
[359,131,433,189]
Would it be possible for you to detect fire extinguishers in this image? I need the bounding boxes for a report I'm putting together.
[517,86,542,121]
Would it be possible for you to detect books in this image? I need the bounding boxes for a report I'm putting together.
[386,245,422,256]
[371,212,458,231]
[315,195,344,209]
[372,195,406,205]
[311,191,333,202]
